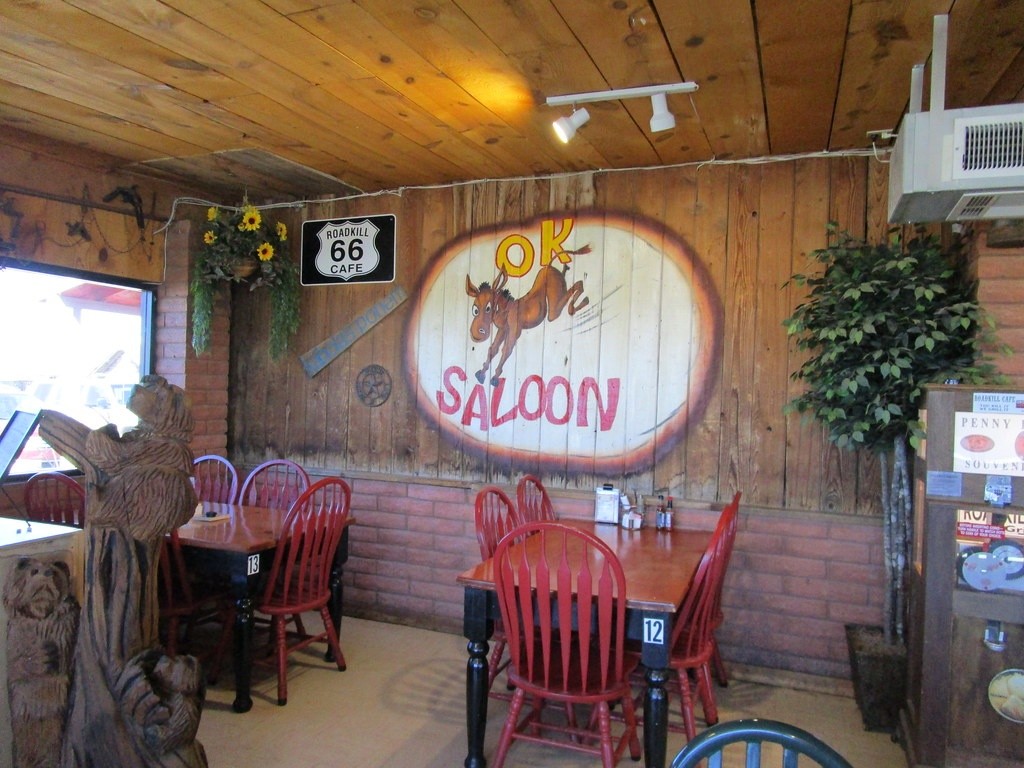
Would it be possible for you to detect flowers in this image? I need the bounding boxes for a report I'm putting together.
[190,201,299,363]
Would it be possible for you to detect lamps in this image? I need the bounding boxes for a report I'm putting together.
[545,80,700,145]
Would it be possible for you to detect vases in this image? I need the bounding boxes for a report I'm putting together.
[215,248,263,283]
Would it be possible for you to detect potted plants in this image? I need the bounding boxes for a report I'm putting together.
[778,219,1016,730]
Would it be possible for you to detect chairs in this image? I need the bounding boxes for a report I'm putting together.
[193,454,240,504]
[671,716,854,768]
[156,528,234,680]
[205,475,352,707]
[475,486,580,744]
[491,521,642,768]
[583,490,745,767]
[517,475,558,540]
[22,470,88,531]
[237,458,311,513]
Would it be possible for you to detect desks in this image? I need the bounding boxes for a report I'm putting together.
[164,502,355,713]
[454,519,716,768]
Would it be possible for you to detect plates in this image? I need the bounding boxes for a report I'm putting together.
[988,668,1024,726]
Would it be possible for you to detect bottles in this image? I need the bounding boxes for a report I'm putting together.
[665,497,674,532]
[637,494,644,514]
[655,495,665,529]
